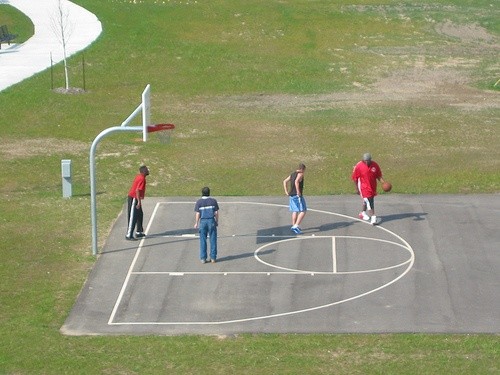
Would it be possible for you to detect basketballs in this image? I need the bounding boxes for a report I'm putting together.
[382,182,392,192]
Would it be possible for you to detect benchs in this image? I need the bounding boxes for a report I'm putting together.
[0,25,19,50]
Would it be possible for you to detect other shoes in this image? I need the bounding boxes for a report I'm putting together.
[126,236,135,240]
[136,234,145,237]
[210,258,216,263]
[201,259,206,263]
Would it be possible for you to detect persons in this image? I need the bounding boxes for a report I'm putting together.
[283,163,307,234]
[352,153,384,224]
[124,165,150,241]
[193,187,220,264]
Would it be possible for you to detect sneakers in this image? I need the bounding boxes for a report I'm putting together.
[370,216,377,225]
[291,226,304,234]
[359,212,370,221]
